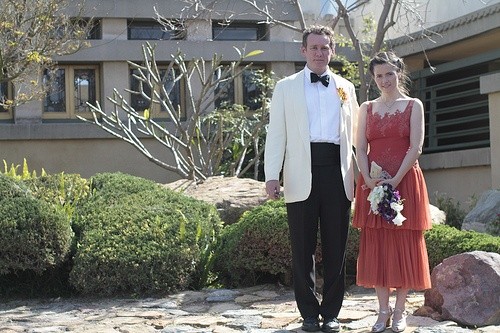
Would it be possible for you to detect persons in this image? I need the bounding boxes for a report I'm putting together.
[264,25,370,333]
[352,51,432,333]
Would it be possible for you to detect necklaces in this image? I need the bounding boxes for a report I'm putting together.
[381,93,401,111]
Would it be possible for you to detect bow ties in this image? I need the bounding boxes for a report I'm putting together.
[310,72,330,88]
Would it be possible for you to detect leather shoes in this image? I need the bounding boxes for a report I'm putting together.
[392,306,407,333]
[321,317,340,333]
[302,314,321,332]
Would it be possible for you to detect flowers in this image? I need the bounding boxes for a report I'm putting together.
[335,87,348,108]
[361,183,407,227]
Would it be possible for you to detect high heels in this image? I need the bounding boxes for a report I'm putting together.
[371,305,393,333]
